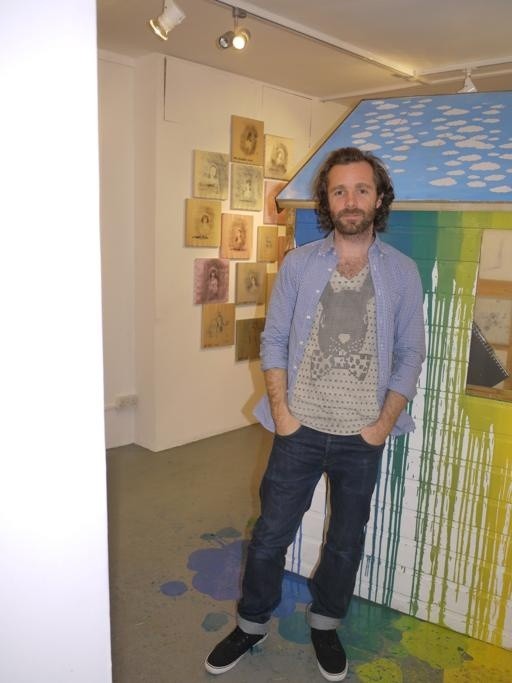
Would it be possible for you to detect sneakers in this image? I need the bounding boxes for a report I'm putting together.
[310,627,349,681]
[204,625,269,674]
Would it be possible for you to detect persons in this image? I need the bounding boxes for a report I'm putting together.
[203,146,426,682]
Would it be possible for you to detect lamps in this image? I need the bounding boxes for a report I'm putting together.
[149,0,250,52]
[455,56,478,94]
